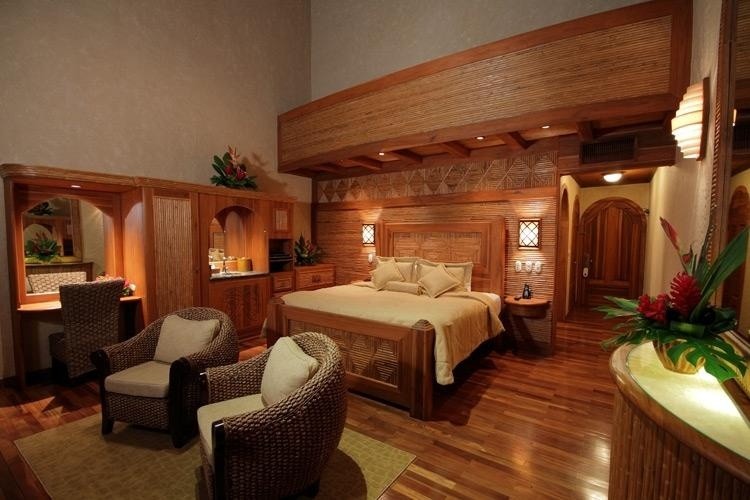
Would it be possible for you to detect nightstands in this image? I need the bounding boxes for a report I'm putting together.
[505,296,549,319]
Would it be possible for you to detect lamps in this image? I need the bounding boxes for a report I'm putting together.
[603,173,622,183]
[518,219,540,250]
[361,223,376,247]
[670,76,710,162]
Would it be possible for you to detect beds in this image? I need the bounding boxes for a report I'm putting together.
[259,216,506,420]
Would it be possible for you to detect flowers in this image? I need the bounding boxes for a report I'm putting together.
[209,143,257,190]
[294,232,322,265]
[594,216,750,382]
[96,273,136,296]
[27,232,62,262]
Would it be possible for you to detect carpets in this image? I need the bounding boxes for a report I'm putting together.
[14,410,416,500]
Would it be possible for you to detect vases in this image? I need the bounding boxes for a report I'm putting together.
[652,336,706,374]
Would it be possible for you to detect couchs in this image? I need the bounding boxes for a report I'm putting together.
[195,331,348,500]
[90,306,240,449]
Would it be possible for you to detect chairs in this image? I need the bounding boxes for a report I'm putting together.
[28,271,87,292]
[48,277,125,382]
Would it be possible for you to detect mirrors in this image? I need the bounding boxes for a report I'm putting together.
[21,196,116,297]
[705,0,750,354]
[23,199,83,263]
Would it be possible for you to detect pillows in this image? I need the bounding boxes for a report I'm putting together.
[261,335,319,404]
[368,255,473,298]
[153,314,220,363]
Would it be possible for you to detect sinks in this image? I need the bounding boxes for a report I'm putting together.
[221,273,242,276]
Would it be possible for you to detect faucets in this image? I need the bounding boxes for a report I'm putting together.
[222,257,227,274]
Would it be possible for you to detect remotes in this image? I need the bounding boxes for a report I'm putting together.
[514,295,521,300]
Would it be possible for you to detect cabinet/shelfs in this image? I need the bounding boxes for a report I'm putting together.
[121,187,201,324]
[295,264,337,291]
[270,270,296,297]
[209,275,273,339]
